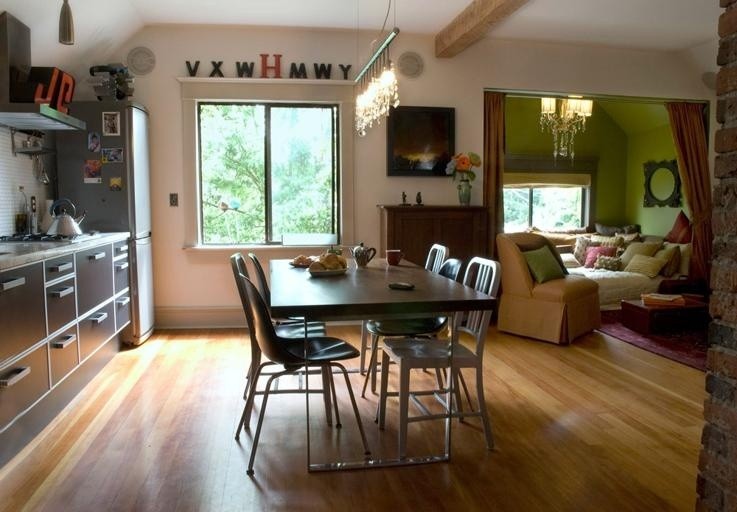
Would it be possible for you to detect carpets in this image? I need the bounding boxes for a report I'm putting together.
[595,316,710,372]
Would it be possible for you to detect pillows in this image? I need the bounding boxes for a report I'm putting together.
[594,254,620,271]
[621,240,663,269]
[585,247,616,269]
[616,234,640,246]
[664,242,689,277]
[597,223,640,238]
[653,246,681,277]
[663,210,692,243]
[521,246,566,284]
[574,234,601,265]
[623,254,667,278]
[558,252,581,268]
[592,235,624,253]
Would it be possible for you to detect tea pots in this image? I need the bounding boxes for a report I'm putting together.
[46,199,87,239]
[350,240,376,269]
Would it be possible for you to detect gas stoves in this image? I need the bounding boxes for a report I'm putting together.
[1,230,100,243]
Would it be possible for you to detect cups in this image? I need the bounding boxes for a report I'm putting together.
[385,249,402,265]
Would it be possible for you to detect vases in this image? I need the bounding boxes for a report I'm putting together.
[457,180,473,206]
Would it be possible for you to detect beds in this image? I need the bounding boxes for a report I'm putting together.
[527,234,694,310]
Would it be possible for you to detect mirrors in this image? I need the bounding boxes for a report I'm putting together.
[643,160,682,208]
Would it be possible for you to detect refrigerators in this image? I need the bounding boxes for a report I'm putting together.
[56,100,155,346]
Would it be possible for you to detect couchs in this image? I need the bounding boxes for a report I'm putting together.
[495,231,602,346]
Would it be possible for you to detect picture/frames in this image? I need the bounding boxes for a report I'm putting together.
[386,107,455,177]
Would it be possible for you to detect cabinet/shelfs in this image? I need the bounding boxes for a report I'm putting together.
[0,261,51,432]
[76,243,115,363]
[375,203,489,325]
[45,253,79,387]
[115,241,132,330]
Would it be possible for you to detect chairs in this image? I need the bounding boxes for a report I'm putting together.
[235,273,372,476]
[231,254,342,439]
[246,253,328,380]
[360,242,451,393]
[361,257,473,424]
[377,257,502,461]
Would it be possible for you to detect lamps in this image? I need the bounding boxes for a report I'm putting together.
[539,97,594,166]
[353,27,401,133]
[59,1,75,46]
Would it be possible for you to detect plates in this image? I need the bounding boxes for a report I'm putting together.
[308,269,347,277]
[289,262,315,268]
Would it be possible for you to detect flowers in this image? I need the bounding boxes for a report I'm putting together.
[445,152,482,181]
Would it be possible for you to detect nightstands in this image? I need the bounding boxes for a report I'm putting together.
[621,299,688,334]
